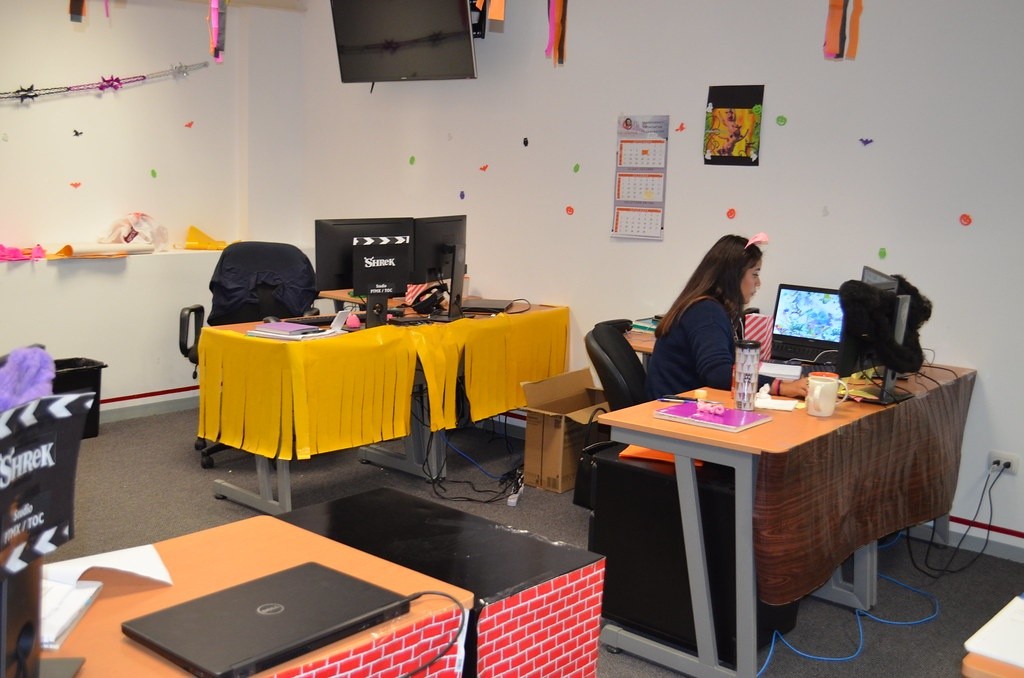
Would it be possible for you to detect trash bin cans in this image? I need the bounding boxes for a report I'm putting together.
[52,357,109,438]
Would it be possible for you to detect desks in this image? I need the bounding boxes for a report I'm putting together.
[602,364,978,677]
[195,289,569,517]
[7,484,608,678]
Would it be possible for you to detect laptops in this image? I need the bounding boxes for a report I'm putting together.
[460,299,512,314]
[121,563,410,678]
[770,284,843,364]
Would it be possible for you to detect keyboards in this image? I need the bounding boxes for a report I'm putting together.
[802,365,837,378]
[284,310,404,326]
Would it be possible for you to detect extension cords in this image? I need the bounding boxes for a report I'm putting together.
[507,484,524,506]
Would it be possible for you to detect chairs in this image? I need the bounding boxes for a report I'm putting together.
[583,317,651,410]
[179,241,319,468]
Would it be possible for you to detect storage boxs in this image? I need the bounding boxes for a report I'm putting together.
[43,356,108,441]
[744,312,773,362]
[513,367,608,494]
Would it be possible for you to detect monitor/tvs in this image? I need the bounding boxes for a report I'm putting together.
[314,217,414,332]
[836,265,911,405]
[330,0,477,84]
[414,215,475,323]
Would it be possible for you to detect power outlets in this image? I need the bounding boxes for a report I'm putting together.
[985,449,1020,475]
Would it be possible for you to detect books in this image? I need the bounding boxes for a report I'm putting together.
[654,402,772,434]
[256,321,319,336]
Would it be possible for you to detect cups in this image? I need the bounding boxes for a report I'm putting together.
[805,372,848,416]
[735,338,760,411]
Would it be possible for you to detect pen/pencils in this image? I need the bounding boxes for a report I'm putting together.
[663,395,697,401]
[657,398,689,403]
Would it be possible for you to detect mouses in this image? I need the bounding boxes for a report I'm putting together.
[263,316,281,323]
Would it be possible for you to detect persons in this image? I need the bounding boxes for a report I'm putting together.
[634,236,811,402]
